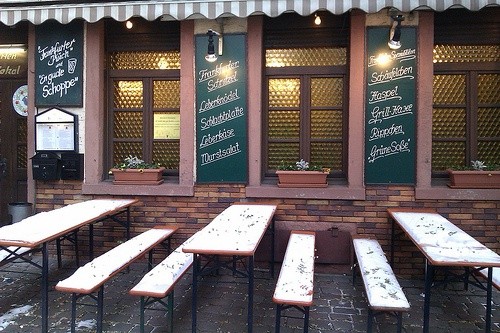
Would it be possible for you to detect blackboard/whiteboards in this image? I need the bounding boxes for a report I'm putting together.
[193,32,249,183]
[35,18,84,108]
[364,24,419,186]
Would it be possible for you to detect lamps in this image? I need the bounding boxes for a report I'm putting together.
[204,18,224,63]
[388,7,405,50]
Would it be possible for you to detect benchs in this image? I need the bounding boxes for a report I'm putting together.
[271,229,316,333]
[0,199,142,333]
[55,224,180,333]
[183,204,279,333]
[129,231,220,333]
[385,205,500,333]
[350,233,412,333]
[474,265,500,291]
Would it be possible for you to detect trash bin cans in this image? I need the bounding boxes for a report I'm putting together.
[8,202,33,225]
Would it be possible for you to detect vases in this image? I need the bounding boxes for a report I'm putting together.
[108,168,166,185]
[276,170,329,188]
[443,170,500,189]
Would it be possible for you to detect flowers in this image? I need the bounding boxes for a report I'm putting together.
[124,154,145,169]
[296,160,310,171]
[470,160,487,170]
